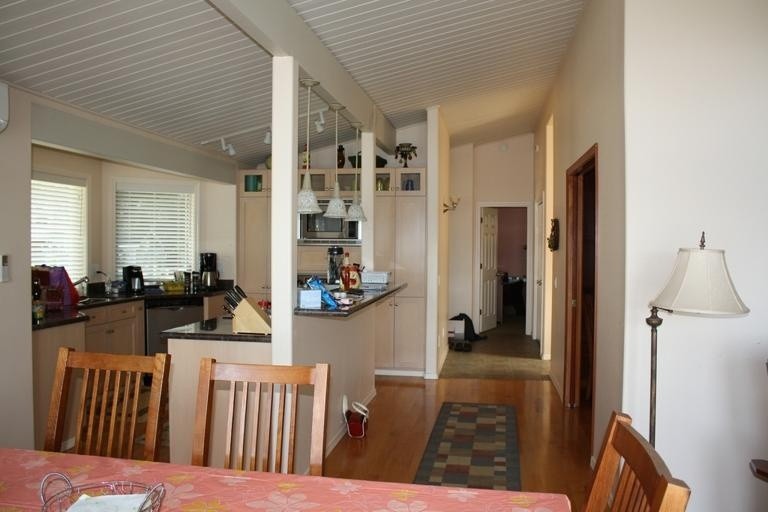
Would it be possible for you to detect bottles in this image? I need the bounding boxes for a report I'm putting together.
[32,282,44,325]
[183,271,200,291]
[302,144,311,169]
[327,247,351,286]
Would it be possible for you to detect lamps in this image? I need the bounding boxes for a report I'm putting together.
[202,108,330,156]
[646,230,750,449]
[321,105,352,217]
[343,123,367,220]
[296,80,322,213]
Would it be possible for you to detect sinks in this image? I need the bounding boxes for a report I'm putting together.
[78,297,114,305]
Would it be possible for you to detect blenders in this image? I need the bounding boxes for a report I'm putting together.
[122,264,145,296]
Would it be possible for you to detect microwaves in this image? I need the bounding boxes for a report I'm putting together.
[298,197,360,240]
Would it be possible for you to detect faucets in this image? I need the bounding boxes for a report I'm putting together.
[72,276,90,286]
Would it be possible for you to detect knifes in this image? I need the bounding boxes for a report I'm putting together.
[221,285,247,314]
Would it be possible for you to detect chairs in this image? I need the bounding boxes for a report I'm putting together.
[46,347,169,463]
[581,410,691,511]
[190,359,331,478]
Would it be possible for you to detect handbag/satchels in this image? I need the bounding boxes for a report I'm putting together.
[340,393,370,438]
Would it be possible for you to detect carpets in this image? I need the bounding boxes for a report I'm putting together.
[438,325,555,380]
[411,401,522,491]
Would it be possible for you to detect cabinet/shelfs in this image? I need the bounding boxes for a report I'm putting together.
[35,166,426,449]
